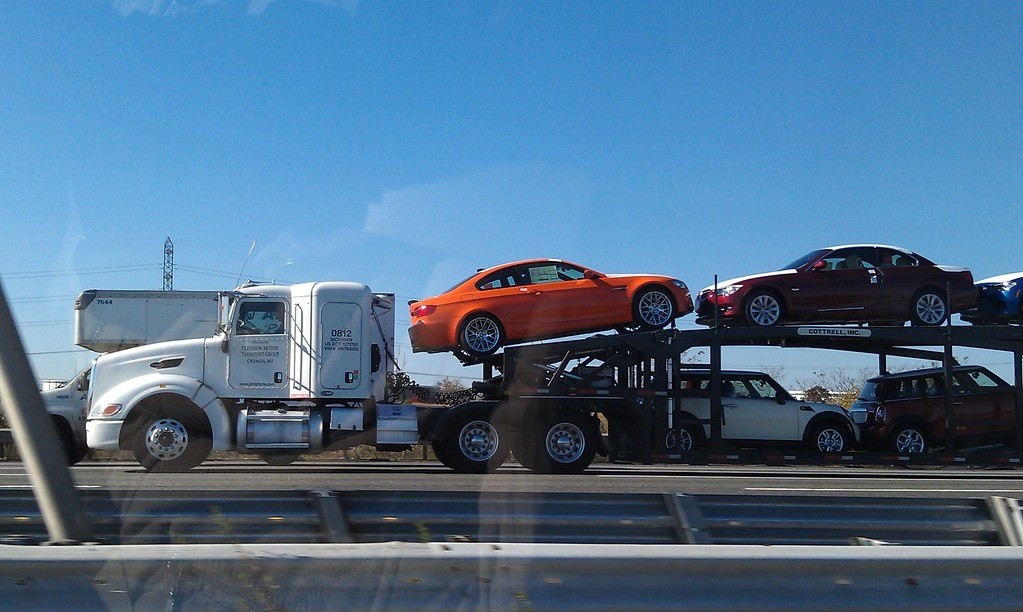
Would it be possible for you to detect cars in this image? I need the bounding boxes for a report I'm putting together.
[851,364,1014,457]
[961,272,1023,323]
[407,258,694,361]
[695,245,978,327]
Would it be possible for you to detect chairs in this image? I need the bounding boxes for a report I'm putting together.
[914,379,930,394]
[896,257,911,266]
[843,254,862,268]
[723,380,736,397]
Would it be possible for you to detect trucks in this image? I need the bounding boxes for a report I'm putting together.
[39,290,232,466]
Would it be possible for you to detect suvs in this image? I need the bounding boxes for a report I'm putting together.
[678,371,856,456]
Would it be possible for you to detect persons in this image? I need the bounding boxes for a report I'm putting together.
[272,311,283,335]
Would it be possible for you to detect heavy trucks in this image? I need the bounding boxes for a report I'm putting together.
[86,274,1023,471]
